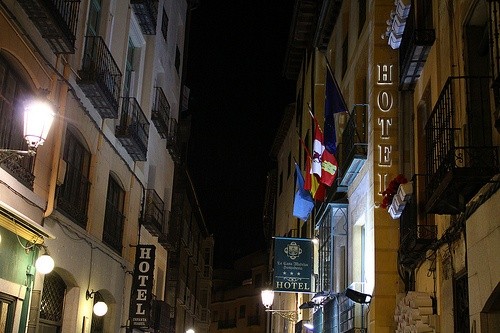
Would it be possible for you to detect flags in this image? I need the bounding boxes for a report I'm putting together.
[324,62,348,155]
[308,118,339,187]
[293,166,315,222]
[301,144,327,202]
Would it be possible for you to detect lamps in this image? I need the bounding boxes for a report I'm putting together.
[260,288,298,323]
[311,292,335,302]
[300,302,322,309]
[344,287,374,304]
[35,243,54,275]
[86,290,108,316]
[0,89,60,167]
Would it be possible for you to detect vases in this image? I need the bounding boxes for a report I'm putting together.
[388,182,414,220]
[387,0,411,49]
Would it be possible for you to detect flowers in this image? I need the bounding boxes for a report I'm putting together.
[380,0,399,39]
[380,175,408,211]
